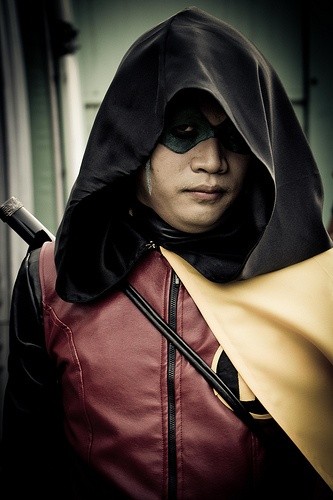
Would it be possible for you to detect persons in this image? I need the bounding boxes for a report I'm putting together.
[0,8,333,500]
